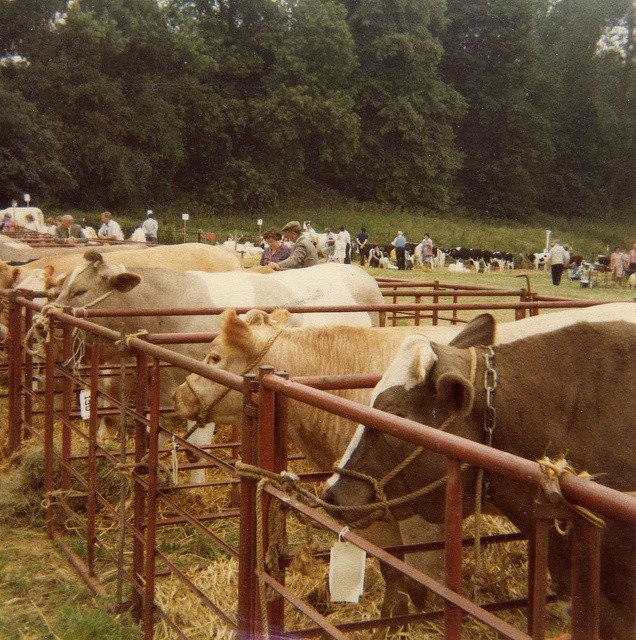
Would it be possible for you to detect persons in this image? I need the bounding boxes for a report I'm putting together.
[547,240,568,287]
[98,212,125,240]
[392,231,405,270]
[306,221,317,244]
[378,247,384,257]
[369,245,380,268]
[25,213,40,233]
[260,227,291,266]
[324,226,335,256]
[356,227,368,266]
[52,214,87,244]
[43,217,55,235]
[142,213,158,243]
[268,221,318,269]
[338,226,351,264]
[420,233,432,272]
[0,213,16,232]
[610,247,624,287]
[628,243,636,274]
[379,251,398,269]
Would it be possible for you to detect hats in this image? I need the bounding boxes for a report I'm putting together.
[282,221,301,231]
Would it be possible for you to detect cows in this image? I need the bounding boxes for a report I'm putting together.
[35,249,388,516]
[0,265,277,441]
[316,311,636,640]
[170,301,635,640]
[356,242,515,274]
[0,244,243,290]
[521,250,552,270]
